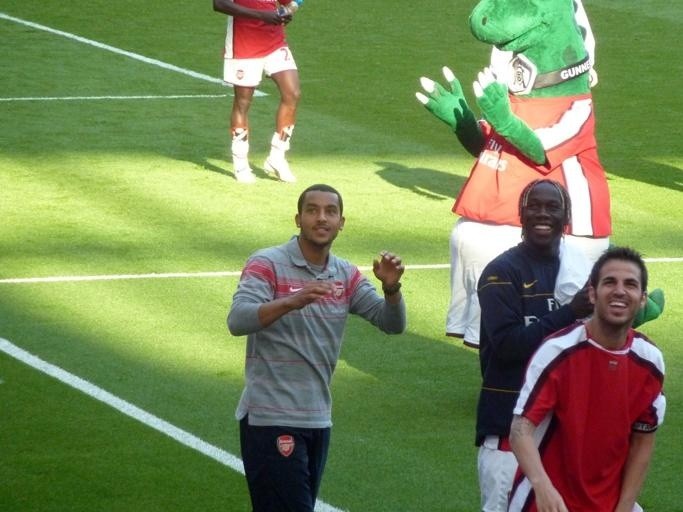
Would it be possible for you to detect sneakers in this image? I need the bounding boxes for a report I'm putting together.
[263,157,298,184]
[233,156,257,185]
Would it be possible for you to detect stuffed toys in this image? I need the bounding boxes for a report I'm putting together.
[412,1,665,347]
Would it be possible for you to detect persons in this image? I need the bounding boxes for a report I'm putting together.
[475,177,595,512]
[211,0,303,184]
[505,243,669,512]
[225,184,408,512]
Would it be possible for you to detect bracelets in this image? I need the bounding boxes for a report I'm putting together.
[379,283,400,298]
